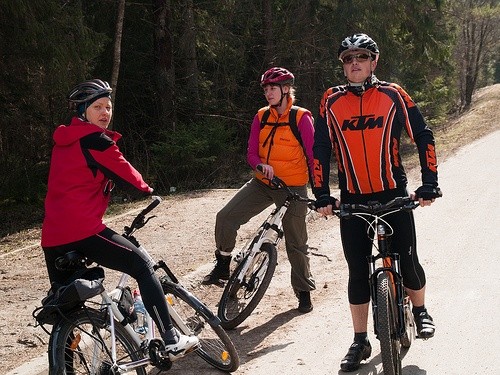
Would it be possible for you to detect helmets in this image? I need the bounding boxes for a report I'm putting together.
[68,79,113,109]
[260,67,296,87]
[337,33,380,59]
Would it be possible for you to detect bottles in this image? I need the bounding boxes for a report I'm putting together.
[132,290,148,334]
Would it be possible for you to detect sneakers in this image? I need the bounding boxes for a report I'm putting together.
[340,337,373,373]
[412,308,436,340]
[165,327,199,361]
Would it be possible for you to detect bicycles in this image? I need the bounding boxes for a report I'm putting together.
[306,190,443,375]
[31,197,241,375]
[216,164,340,331]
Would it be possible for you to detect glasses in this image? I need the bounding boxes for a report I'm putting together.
[342,53,374,64]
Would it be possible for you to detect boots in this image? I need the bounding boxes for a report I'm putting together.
[297,291,314,314]
[202,250,233,286]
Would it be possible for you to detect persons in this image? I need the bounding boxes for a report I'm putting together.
[201,66,316,313]
[41,80,199,375]
[313,33,438,372]
